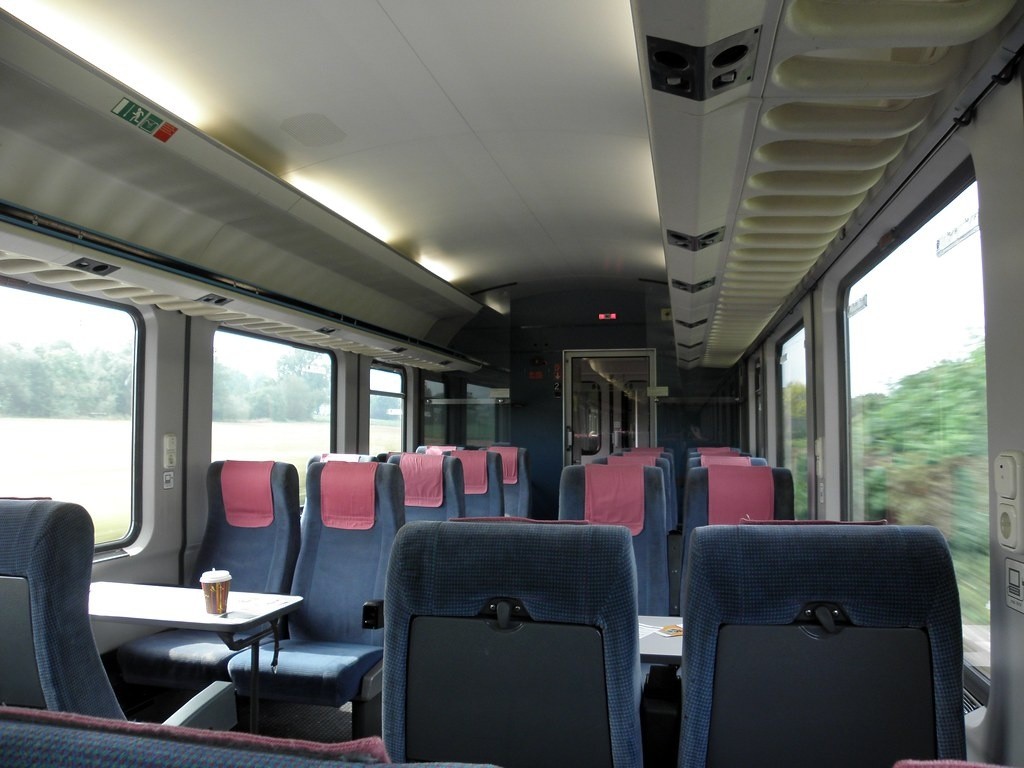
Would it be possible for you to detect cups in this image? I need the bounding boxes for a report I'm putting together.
[199,570,232,614]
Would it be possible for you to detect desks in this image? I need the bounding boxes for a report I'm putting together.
[637,614,685,664]
[88,581,304,735]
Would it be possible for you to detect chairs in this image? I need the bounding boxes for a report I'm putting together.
[612,452,677,533]
[677,525,969,768]
[557,465,669,616]
[0,496,237,732]
[478,446,537,519]
[441,451,505,517]
[228,461,408,741]
[387,453,466,522]
[681,467,794,618]
[592,456,673,606]
[115,461,302,682]
[686,455,768,468]
[380,520,644,768]
[301,452,379,530]
